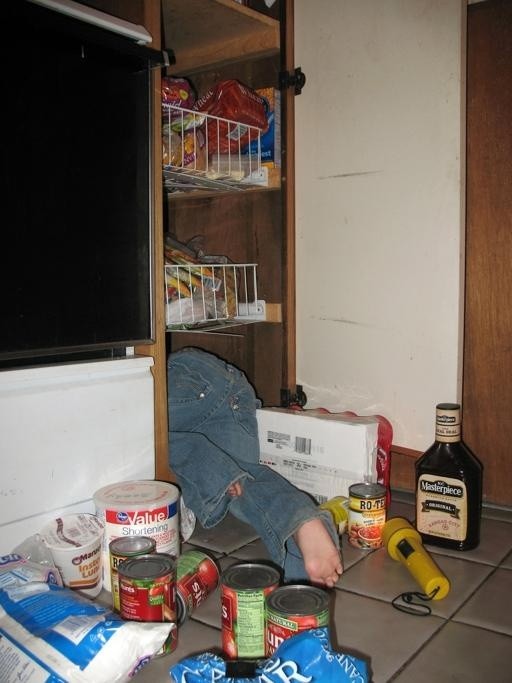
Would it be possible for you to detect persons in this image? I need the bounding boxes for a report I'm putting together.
[164,345,343,591]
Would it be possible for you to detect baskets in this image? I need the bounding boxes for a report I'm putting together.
[164,257,262,334]
[162,102,268,196]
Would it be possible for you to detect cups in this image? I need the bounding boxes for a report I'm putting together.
[40,512,105,599]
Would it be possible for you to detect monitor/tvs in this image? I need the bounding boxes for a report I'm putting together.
[0,0,157,358]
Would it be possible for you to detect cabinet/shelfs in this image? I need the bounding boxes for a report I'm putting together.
[161,0,297,515]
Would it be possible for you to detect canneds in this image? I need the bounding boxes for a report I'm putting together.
[173,550,220,629]
[119,553,176,659]
[222,562,278,660]
[264,584,330,657]
[109,536,158,613]
[349,483,387,549]
[318,496,351,539]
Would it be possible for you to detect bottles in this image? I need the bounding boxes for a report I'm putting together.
[415,402,485,552]
